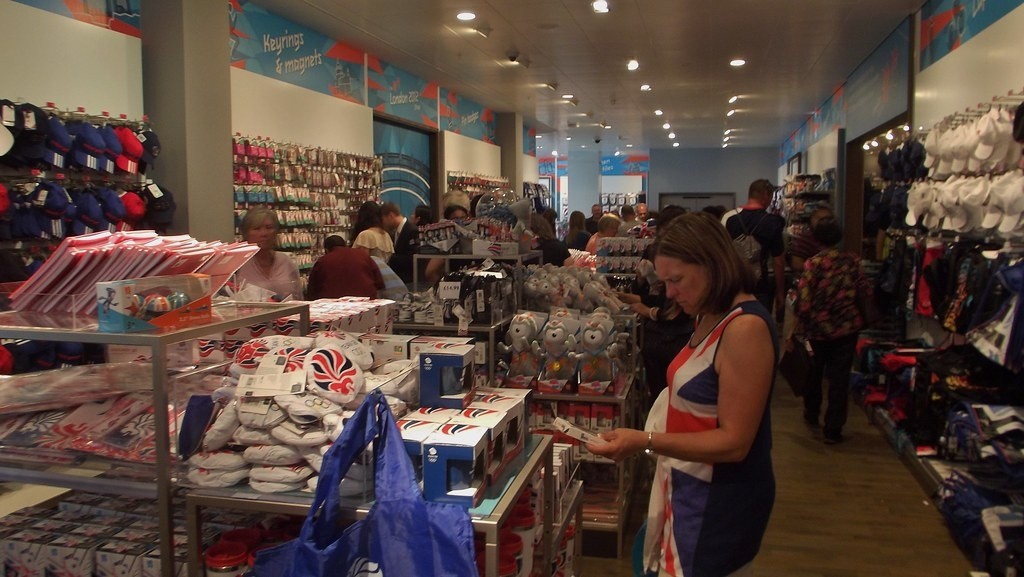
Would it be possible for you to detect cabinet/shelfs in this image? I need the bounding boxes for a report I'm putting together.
[391,254,643,577]
[0,302,310,577]
[186,434,586,577]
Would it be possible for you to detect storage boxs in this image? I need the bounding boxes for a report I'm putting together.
[310,330,527,506]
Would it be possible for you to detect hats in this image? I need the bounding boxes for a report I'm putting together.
[0,101,160,174]
[0,339,104,375]
[864,109,1024,235]
[0,181,178,243]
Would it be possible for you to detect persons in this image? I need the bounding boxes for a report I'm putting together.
[410,204,431,227]
[349,200,396,264]
[233,207,306,301]
[423,177,728,404]
[306,235,385,301]
[369,255,409,301]
[379,201,424,287]
[719,179,785,338]
[585,211,779,577]
[790,206,836,298]
[786,216,877,445]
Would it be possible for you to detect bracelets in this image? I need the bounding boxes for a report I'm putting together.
[649,306,660,321]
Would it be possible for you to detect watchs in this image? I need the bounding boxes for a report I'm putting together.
[644,431,655,456]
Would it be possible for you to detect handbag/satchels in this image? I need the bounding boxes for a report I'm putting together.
[780,320,820,398]
[640,321,694,358]
[644,387,670,462]
[859,268,880,310]
[243,387,482,577]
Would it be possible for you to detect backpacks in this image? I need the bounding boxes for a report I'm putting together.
[733,207,770,284]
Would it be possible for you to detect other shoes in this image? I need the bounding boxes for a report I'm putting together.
[805,416,818,425]
[824,435,842,443]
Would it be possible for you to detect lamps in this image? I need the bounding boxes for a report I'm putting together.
[518,56,531,69]
[547,81,559,91]
[476,23,493,38]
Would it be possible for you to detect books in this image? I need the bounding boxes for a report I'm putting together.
[8,229,262,316]
